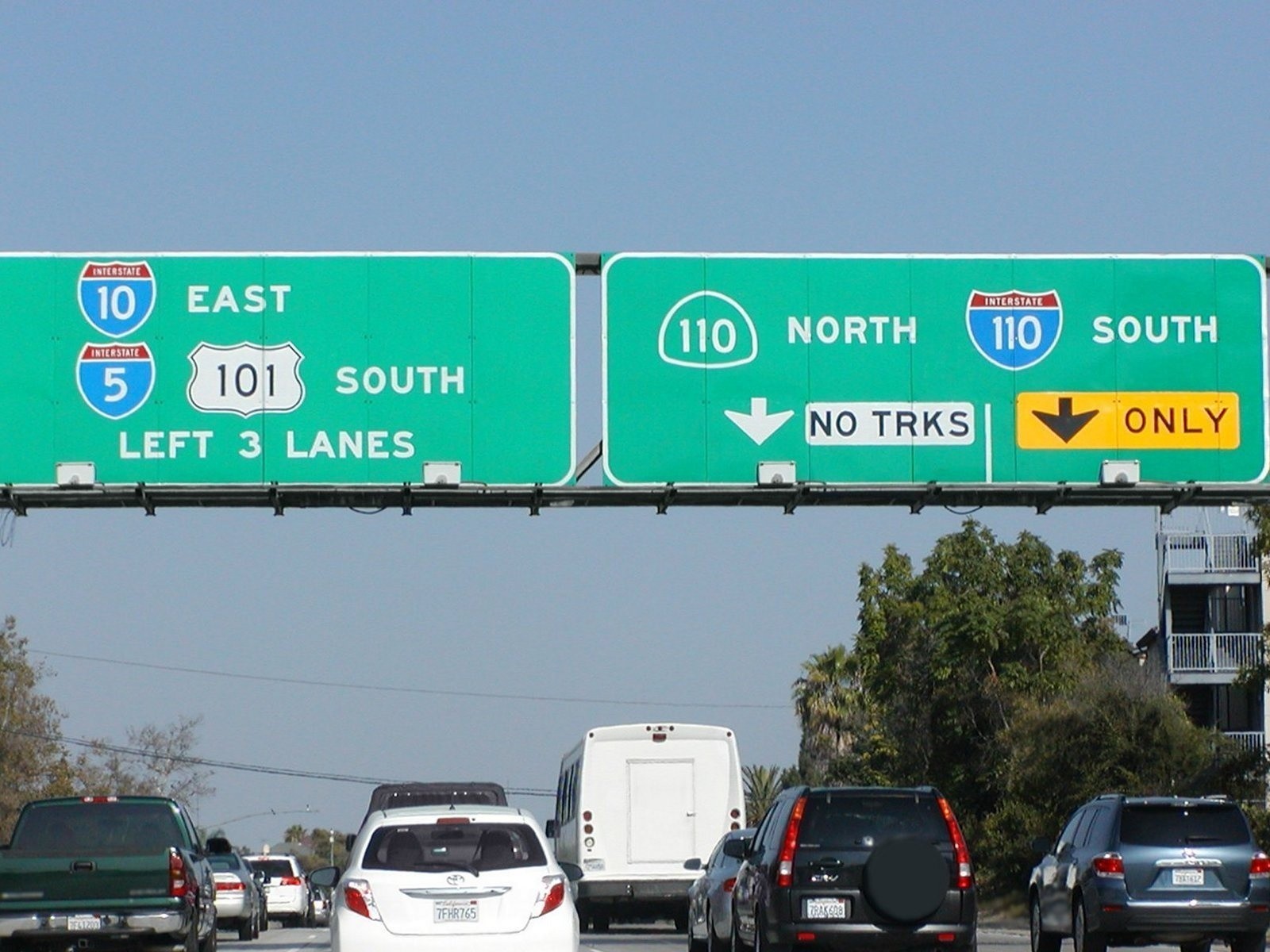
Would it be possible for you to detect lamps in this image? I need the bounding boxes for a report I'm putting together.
[1099,460,1140,486]
[56,462,95,488]
[757,461,796,488]
[423,461,461,487]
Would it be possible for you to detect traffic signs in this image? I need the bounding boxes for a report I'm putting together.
[601,251,1269,491]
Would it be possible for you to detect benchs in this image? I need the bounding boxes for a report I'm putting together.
[384,831,514,868]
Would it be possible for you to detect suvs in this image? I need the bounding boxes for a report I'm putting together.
[1026,791,1269,951]
[722,785,980,952]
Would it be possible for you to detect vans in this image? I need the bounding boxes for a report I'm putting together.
[545,723,749,934]
[344,783,508,853]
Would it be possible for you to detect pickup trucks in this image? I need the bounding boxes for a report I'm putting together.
[0,794,233,952]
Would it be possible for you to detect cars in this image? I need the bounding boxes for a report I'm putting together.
[309,804,583,951]
[207,848,332,941]
[686,827,758,952]
